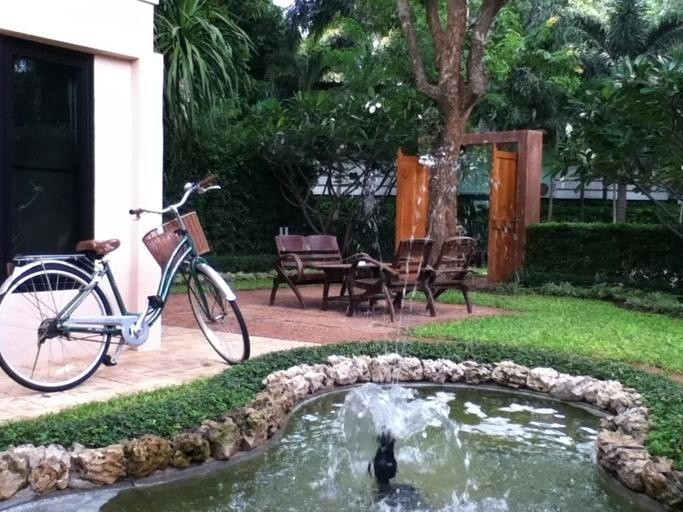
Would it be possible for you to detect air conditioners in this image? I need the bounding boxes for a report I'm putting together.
[539,180,556,198]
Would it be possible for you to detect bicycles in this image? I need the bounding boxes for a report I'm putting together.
[0,174,256,394]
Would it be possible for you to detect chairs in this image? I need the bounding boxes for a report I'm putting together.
[273,233,479,323]
